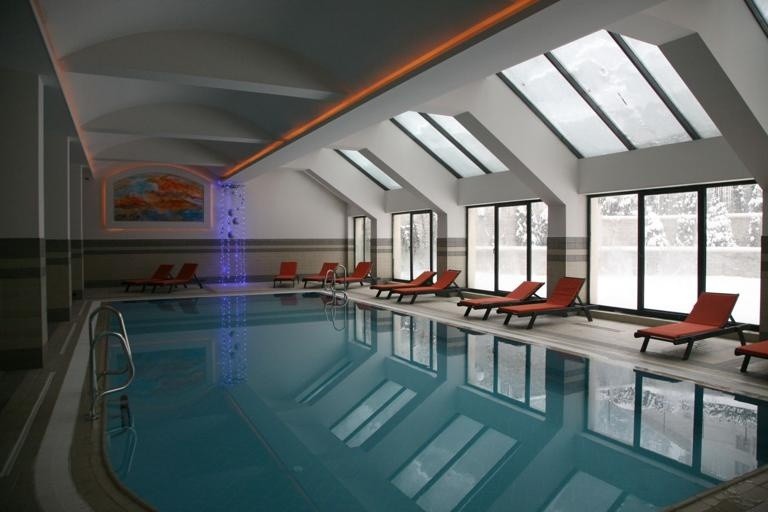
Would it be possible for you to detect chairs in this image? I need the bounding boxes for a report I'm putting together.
[123,262,203,294]
[633,291,768,373]
[273,260,373,289]
[369,268,598,329]
